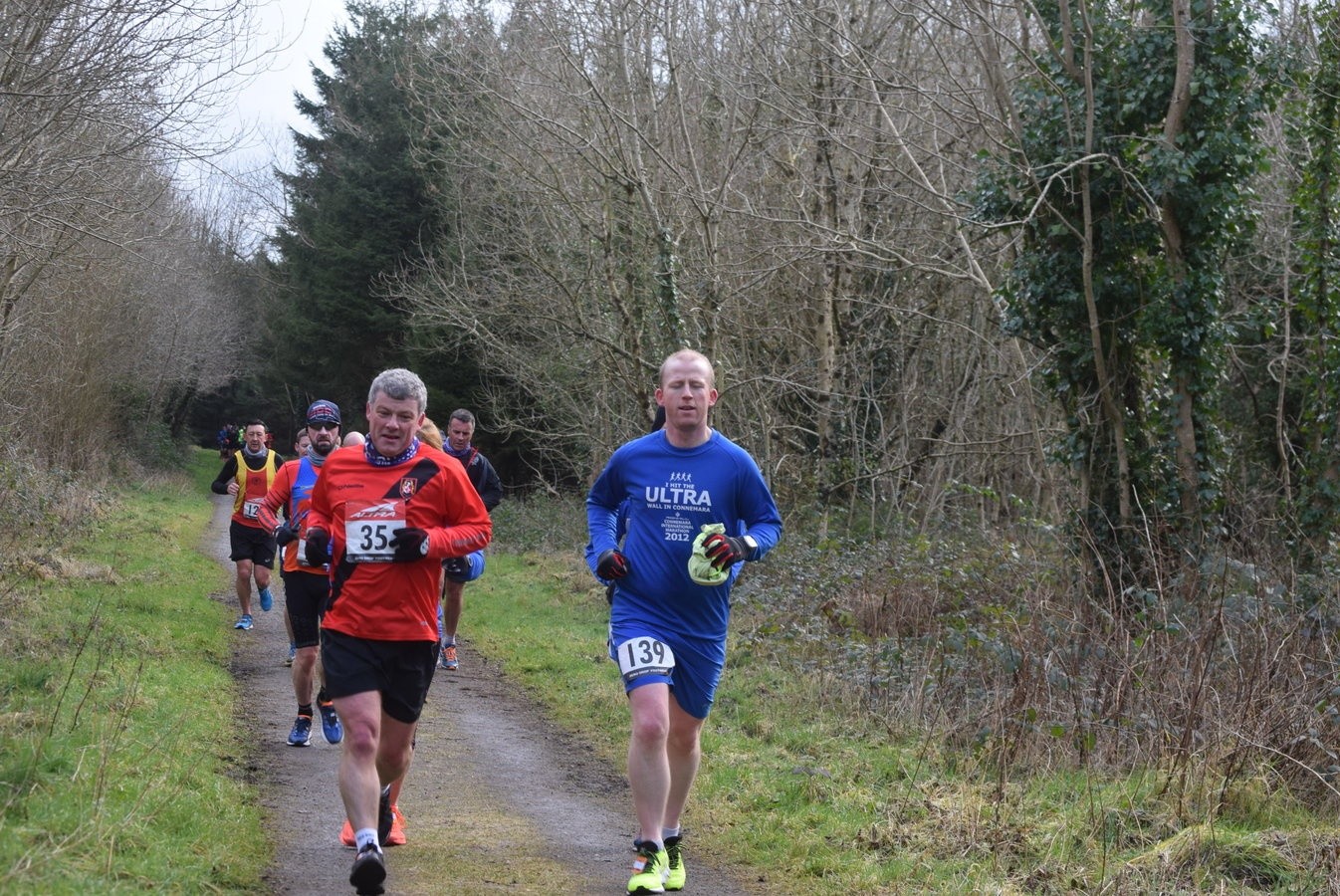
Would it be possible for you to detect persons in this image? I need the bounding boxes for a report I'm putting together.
[304,367,494,896]
[256,398,357,747]
[437,408,502,672]
[218,422,273,463]
[338,417,445,849]
[341,431,365,448]
[582,347,784,896]
[210,418,287,630]
[280,426,313,667]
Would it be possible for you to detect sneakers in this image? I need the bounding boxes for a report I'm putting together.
[340,819,357,846]
[285,715,312,746]
[284,643,297,666]
[627,836,670,895]
[386,803,407,844]
[234,614,253,629]
[257,587,273,611]
[351,837,386,895]
[380,787,394,846]
[316,694,345,744]
[663,834,686,890]
[439,641,459,669]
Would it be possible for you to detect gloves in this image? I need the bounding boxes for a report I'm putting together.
[306,527,333,568]
[595,549,631,582]
[272,526,299,547]
[390,528,431,563]
[701,532,751,574]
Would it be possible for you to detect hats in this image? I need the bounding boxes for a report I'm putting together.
[305,399,342,426]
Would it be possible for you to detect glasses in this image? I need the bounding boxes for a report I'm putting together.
[308,422,338,430]
